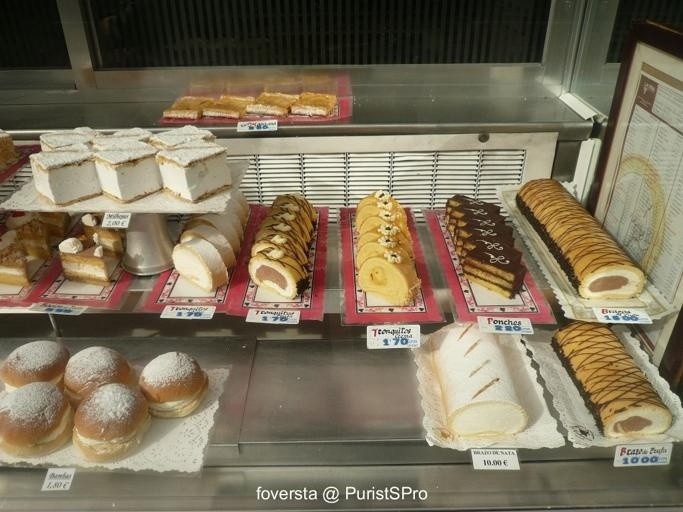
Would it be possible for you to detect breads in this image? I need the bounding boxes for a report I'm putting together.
[0,132,17,171]
[356,191,422,308]
[172,193,317,299]
[516,178,646,299]
[443,194,527,299]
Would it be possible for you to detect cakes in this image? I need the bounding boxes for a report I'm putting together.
[28,123,232,207]
[0,213,125,287]
[552,321,672,438]
[0,339,209,462]
[430,322,527,436]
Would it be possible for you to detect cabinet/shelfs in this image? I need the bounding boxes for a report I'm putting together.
[0,222,683,469]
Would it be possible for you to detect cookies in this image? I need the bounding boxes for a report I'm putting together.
[162,91,338,121]
